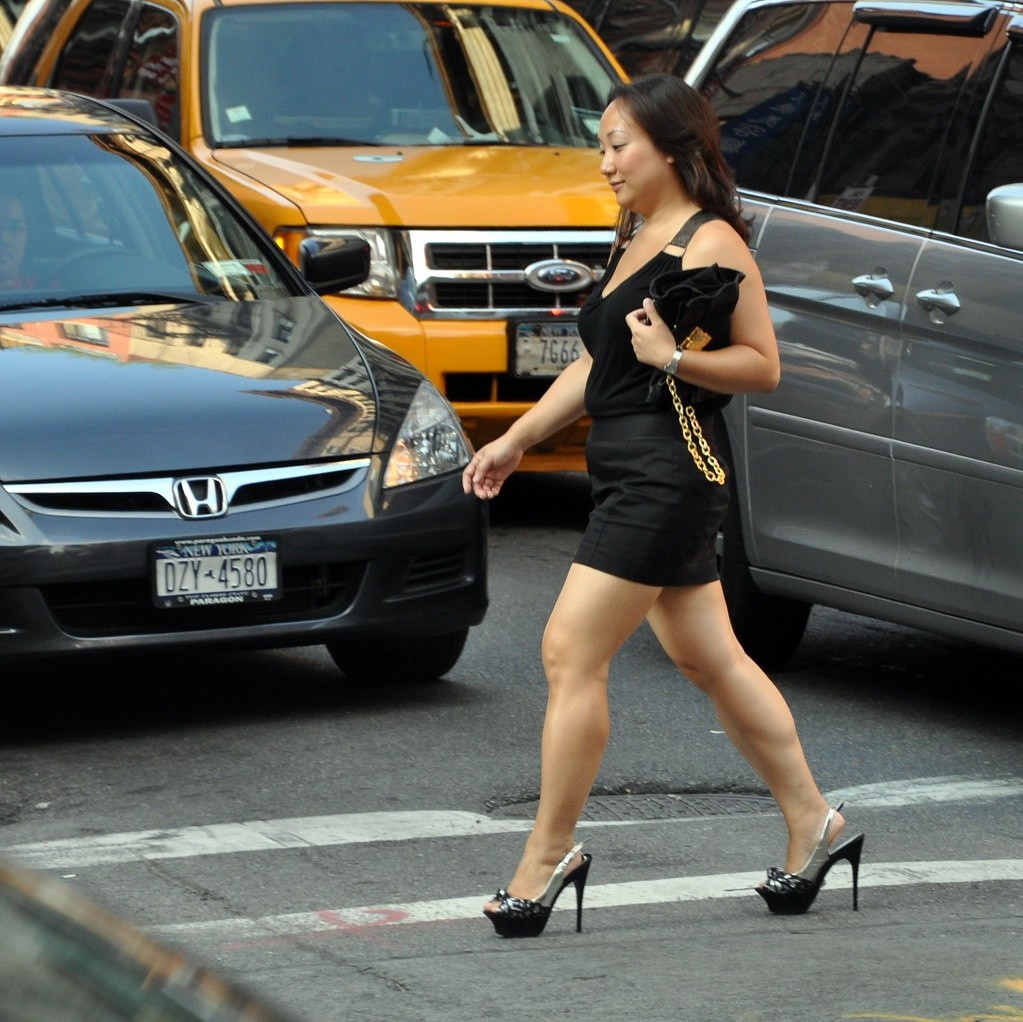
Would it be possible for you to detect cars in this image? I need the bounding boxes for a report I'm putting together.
[2,90,490,742]
[3,0,634,474]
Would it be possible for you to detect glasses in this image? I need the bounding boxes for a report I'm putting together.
[0,218,28,237]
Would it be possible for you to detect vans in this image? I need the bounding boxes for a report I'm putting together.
[687,0,1022,683]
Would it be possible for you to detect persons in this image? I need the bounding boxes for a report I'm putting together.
[464,83,865,937]
[0,193,57,290]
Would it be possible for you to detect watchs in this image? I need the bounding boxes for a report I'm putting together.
[663,348,682,374]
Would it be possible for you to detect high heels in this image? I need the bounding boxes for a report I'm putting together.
[755,802,864,917]
[482,839,592,939]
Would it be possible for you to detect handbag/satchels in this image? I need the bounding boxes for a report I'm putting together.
[647,264,746,485]
